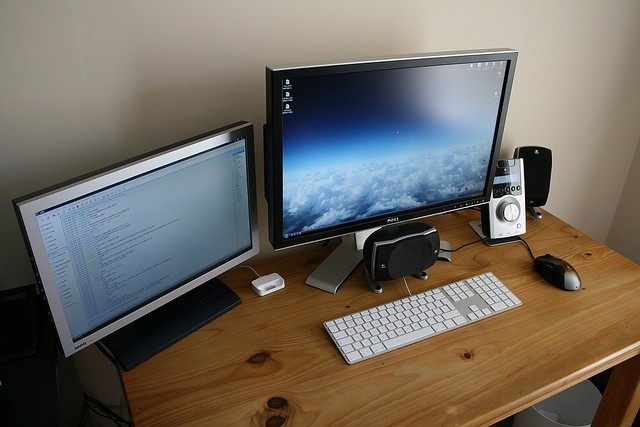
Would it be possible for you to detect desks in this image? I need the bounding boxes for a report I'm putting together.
[117,201,640,426]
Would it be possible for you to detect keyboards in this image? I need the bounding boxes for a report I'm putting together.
[311,271,523,366]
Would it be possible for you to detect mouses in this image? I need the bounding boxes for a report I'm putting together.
[534,252,583,290]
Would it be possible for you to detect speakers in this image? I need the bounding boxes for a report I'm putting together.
[360,221,441,293]
[514,145,553,218]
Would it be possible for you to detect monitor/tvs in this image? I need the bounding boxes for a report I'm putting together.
[264,47,519,294]
[12,121,260,373]
